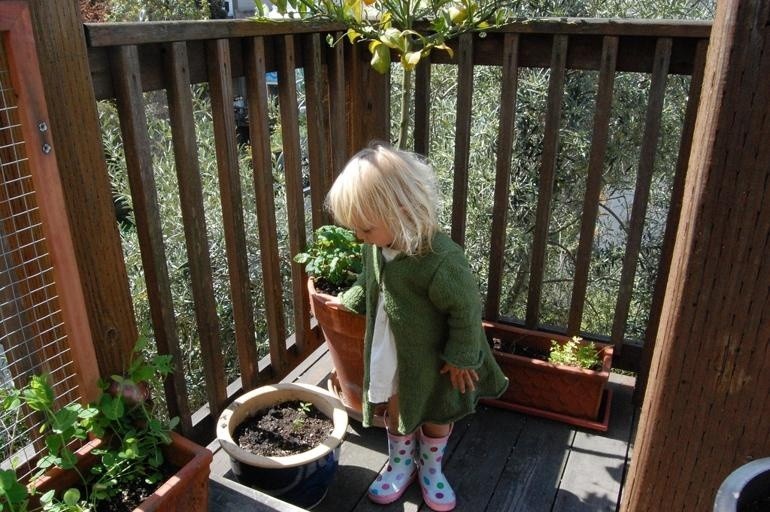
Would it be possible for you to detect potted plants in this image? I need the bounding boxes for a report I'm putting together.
[293,225,401,428]
[473,321,615,431]
[0,313,212,512]
[216,383,348,510]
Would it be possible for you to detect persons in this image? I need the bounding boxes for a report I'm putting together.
[324,140,510,511]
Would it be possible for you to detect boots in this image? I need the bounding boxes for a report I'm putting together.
[367,409,417,505]
[417,421,457,512]
